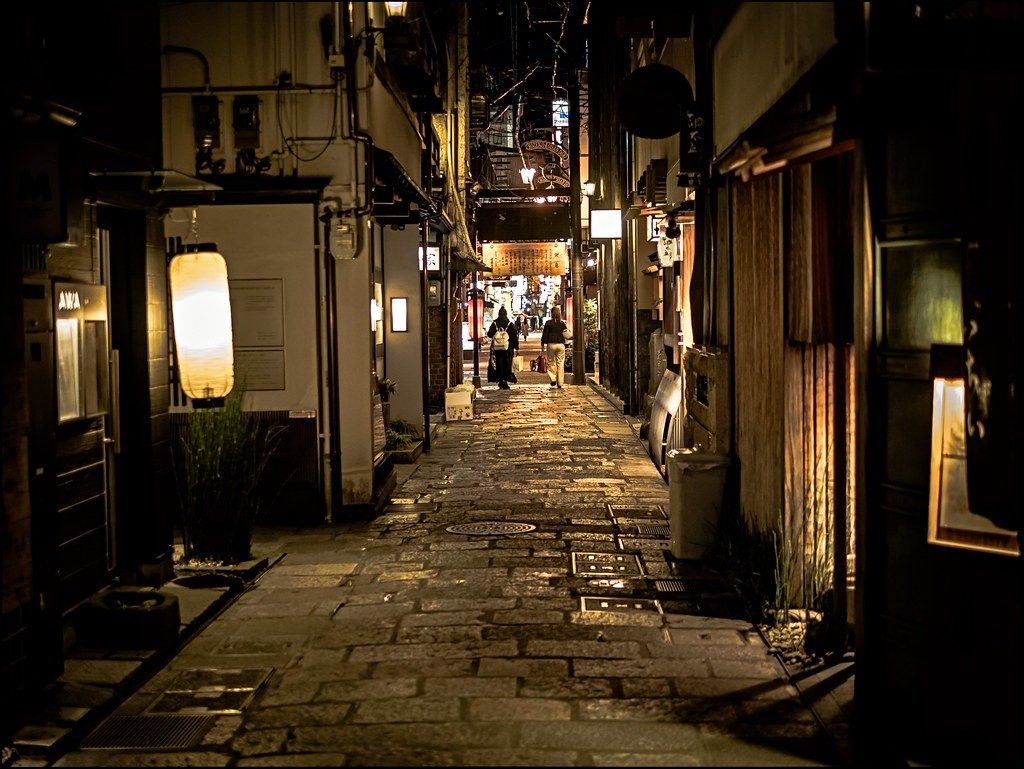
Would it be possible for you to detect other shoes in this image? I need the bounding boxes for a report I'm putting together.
[498,381,510,390]
[551,381,556,386]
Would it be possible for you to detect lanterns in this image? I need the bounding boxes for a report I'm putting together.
[168,242,234,410]
[657,234,677,268]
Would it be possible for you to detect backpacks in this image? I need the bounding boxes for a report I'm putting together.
[493,321,512,350]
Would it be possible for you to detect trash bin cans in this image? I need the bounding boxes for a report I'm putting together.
[664,451,728,569]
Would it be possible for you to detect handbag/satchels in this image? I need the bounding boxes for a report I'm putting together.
[537,349,547,373]
[512,354,524,373]
[562,322,571,339]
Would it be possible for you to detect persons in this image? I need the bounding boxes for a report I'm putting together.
[515,316,529,341]
[531,314,537,331]
[538,313,544,328]
[487,306,519,389]
[541,307,567,388]
